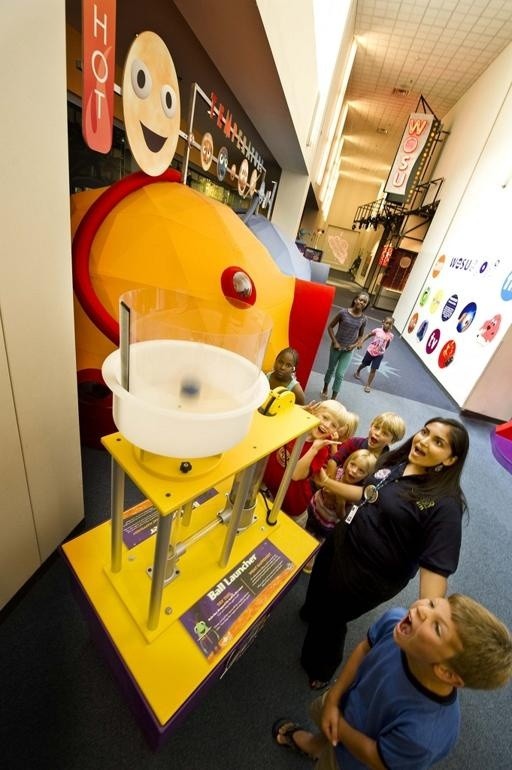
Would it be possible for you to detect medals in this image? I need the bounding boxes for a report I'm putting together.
[363,484,378,504]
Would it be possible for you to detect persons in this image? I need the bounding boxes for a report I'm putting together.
[305,448,377,540]
[330,412,358,457]
[350,254,361,281]
[354,315,395,393]
[269,592,512,770]
[298,415,469,689]
[323,410,407,509]
[258,400,349,528]
[264,346,305,407]
[320,290,371,400]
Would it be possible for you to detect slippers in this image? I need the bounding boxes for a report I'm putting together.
[272,718,322,757]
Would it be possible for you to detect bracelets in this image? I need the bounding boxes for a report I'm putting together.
[321,476,329,487]
